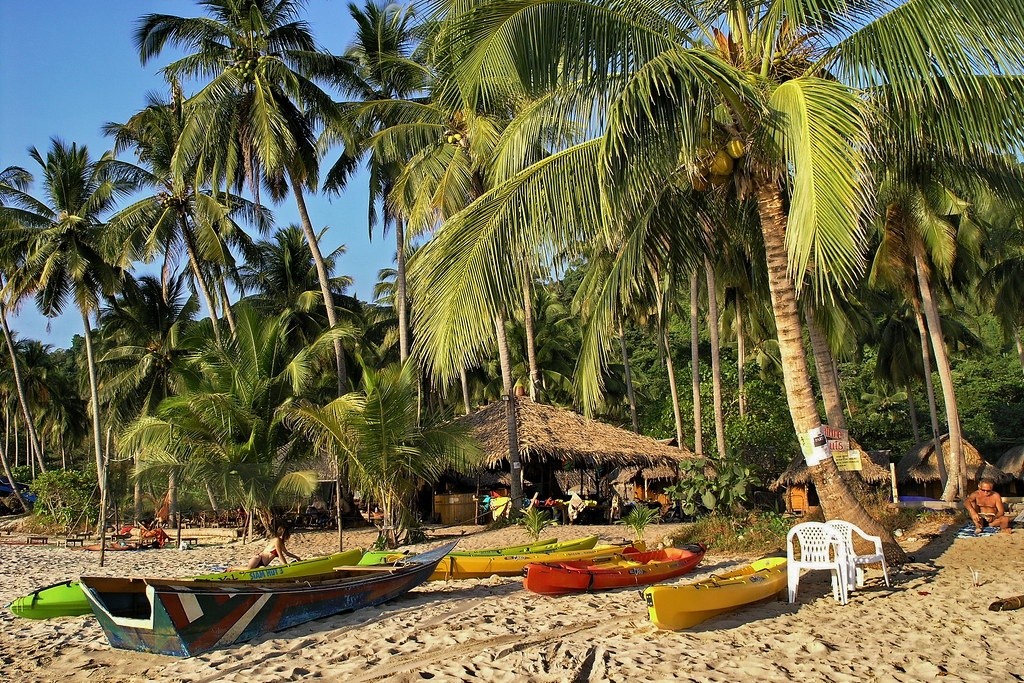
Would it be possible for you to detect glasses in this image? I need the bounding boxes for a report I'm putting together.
[981,488,992,493]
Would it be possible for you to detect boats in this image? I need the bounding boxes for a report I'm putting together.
[10,546,367,620]
[358,533,603,566]
[384,538,646,581]
[638,548,790,631]
[521,542,708,597]
[78,538,461,658]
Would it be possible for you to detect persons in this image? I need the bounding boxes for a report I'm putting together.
[963,478,1012,534]
[248,526,302,571]
[287,494,350,529]
[140,519,176,549]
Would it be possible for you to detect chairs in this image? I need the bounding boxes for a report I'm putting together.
[824,520,890,592]
[786,522,848,606]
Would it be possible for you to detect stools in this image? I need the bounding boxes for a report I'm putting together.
[26,532,198,550]
